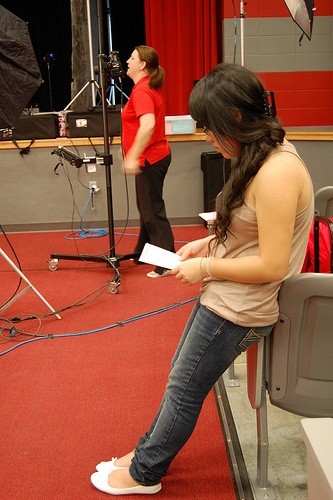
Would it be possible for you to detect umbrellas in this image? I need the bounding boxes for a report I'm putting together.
[0,5,42,129]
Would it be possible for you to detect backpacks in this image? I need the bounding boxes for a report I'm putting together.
[300,216,333,275]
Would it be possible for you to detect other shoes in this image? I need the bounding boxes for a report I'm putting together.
[90,469,162,495]
[96,457,131,472]
[147,268,173,278]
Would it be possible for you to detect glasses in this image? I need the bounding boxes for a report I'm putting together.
[201,127,211,136]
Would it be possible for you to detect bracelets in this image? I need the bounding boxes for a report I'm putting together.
[206,256,213,277]
[199,256,206,277]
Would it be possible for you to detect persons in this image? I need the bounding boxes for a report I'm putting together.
[89,63,314,495]
[121,45,179,278]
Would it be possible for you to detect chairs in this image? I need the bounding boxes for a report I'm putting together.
[315,184,333,223]
[246,272,333,490]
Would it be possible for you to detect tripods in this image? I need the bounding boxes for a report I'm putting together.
[48,1,146,282]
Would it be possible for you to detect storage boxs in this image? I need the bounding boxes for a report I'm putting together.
[11,114,62,139]
[164,113,198,136]
[65,111,122,137]
